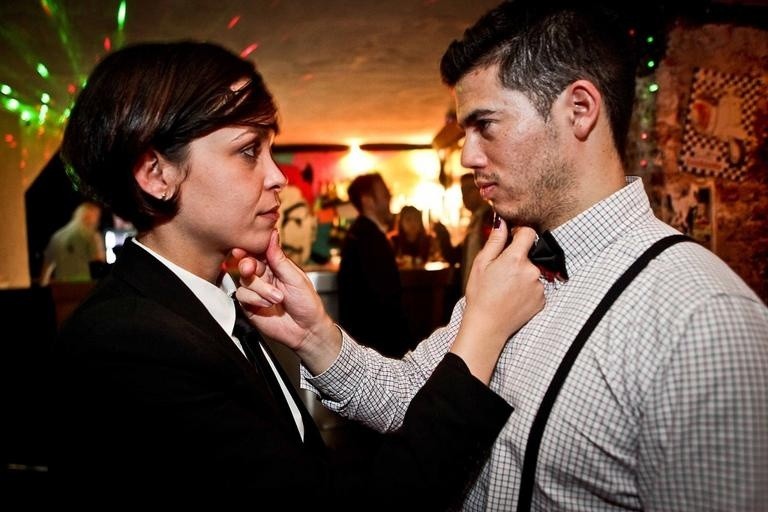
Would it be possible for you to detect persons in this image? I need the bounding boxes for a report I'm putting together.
[334,172,405,477]
[41,204,108,286]
[388,205,434,264]
[431,173,493,312]
[42,38,546,511]
[231,1,768,512]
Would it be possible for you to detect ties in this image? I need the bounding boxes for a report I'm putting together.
[233,301,270,375]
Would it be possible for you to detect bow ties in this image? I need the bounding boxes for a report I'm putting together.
[526,230,569,282]
